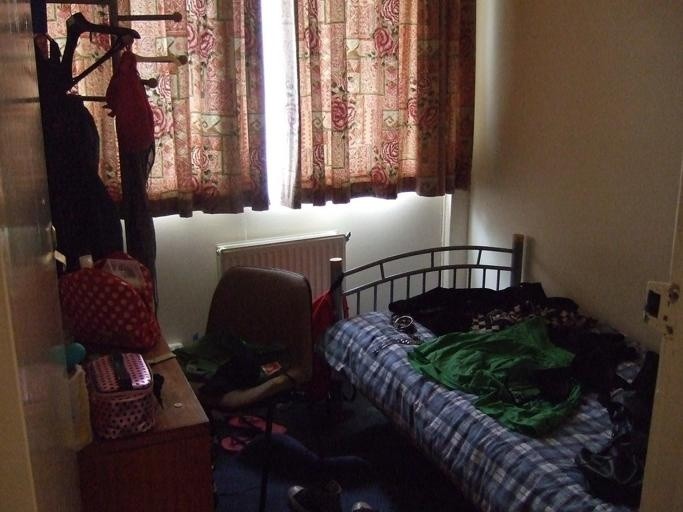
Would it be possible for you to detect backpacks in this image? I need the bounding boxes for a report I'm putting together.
[302,290,356,404]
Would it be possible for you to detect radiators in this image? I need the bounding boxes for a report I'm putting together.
[217,232,347,358]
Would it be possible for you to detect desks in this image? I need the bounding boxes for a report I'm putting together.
[77,329,216,512]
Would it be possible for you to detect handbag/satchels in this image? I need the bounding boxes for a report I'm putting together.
[58,251,161,351]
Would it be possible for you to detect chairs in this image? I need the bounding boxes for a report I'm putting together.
[192,266,315,510]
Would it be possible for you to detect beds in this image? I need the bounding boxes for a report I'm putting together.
[329,233,665,509]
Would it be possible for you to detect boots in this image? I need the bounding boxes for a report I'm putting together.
[287,478,372,512]
